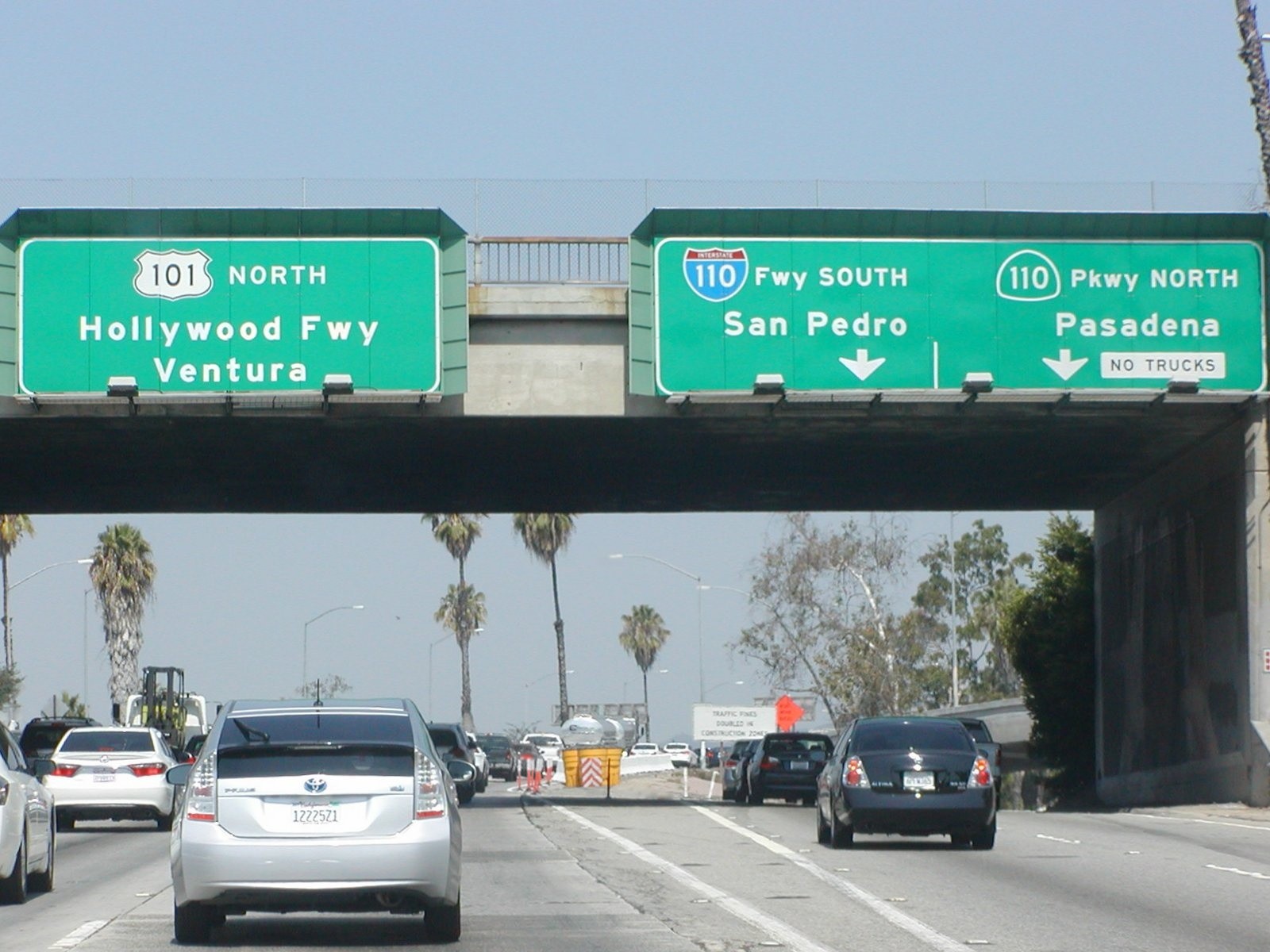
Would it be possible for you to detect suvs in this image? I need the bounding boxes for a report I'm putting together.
[741,731,835,804]
[524,733,566,771]
[19,715,103,785]
[475,734,521,782]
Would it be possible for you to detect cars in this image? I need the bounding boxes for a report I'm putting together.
[629,743,663,758]
[0,725,57,903]
[722,738,754,800]
[45,724,184,831]
[730,738,808,803]
[512,742,545,777]
[466,733,490,792]
[425,721,483,807]
[817,716,998,852]
[693,745,721,768]
[163,693,463,945]
[663,743,699,768]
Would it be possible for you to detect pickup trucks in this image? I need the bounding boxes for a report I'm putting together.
[950,718,1003,812]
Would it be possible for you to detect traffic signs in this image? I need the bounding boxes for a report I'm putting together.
[649,230,1268,398]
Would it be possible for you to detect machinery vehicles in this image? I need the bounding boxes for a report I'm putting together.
[125,664,208,760]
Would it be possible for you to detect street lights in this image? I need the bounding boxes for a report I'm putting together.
[304,604,365,693]
[2,558,96,725]
[623,668,670,706]
[609,553,745,768]
[428,627,483,721]
